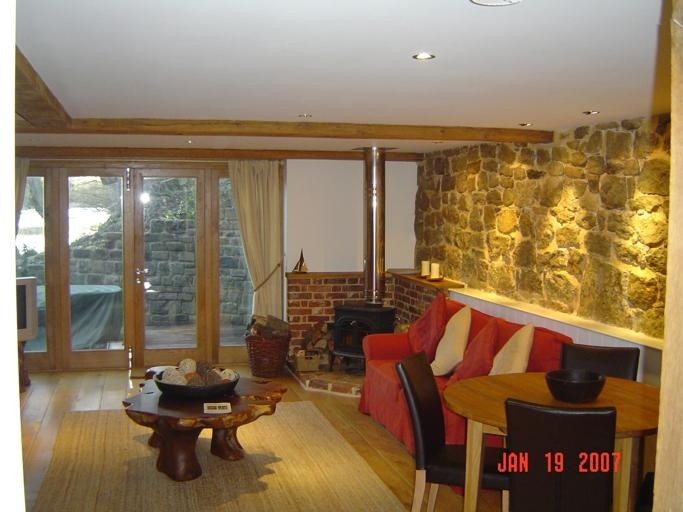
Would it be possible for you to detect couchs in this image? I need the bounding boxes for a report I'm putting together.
[357,289,575,498]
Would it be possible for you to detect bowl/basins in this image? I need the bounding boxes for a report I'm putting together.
[544,370,606,402]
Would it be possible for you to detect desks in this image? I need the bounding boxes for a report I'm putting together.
[441,377,660,512]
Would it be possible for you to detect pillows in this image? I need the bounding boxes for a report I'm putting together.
[445,319,499,385]
[484,320,539,377]
[427,306,474,377]
[407,288,447,361]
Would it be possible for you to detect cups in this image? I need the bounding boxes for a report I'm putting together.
[429,263,440,279]
[421,261,430,276]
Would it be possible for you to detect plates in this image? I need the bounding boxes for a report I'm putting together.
[152,369,239,398]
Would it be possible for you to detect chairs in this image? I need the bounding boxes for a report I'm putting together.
[502,396,617,511]
[558,340,640,386]
[393,350,513,511]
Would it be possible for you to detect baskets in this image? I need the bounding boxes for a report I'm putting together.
[246,334,291,377]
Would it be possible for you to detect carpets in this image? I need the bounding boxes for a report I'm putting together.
[32,400,407,511]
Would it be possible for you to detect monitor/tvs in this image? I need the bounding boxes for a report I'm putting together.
[17,276,38,341]
